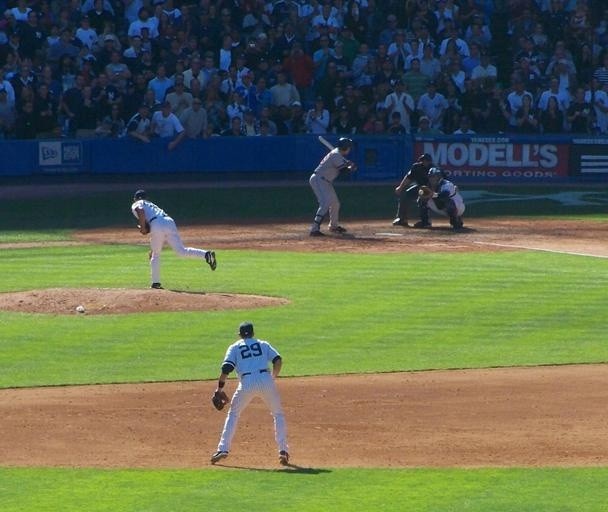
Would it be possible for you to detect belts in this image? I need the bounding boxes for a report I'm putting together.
[450,191,456,198]
[314,172,331,183]
[243,369,267,375]
[149,214,165,222]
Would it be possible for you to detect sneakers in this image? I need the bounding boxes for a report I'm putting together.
[206,251,216,270]
[415,221,431,228]
[211,451,226,464]
[152,283,162,289]
[453,224,463,233]
[309,231,325,236]
[328,226,346,232]
[393,218,407,225]
[279,451,288,465]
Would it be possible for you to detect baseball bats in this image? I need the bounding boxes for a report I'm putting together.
[319,136,358,171]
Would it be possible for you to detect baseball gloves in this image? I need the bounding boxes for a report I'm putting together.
[137,222,149,235]
[420,185,434,201]
[212,391,229,411]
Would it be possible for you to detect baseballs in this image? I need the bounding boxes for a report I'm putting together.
[419,189,425,195]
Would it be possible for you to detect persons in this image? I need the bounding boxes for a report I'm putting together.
[414,167,466,228]
[393,154,444,226]
[212,320,289,465]
[309,138,357,235]
[1,1,608,148]
[131,190,216,289]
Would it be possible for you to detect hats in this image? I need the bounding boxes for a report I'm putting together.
[240,322,253,335]
[418,154,432,161]
[134,190,146,198]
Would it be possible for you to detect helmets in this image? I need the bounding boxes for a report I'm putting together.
[339,137,352,147]
[428,167,444,176]
